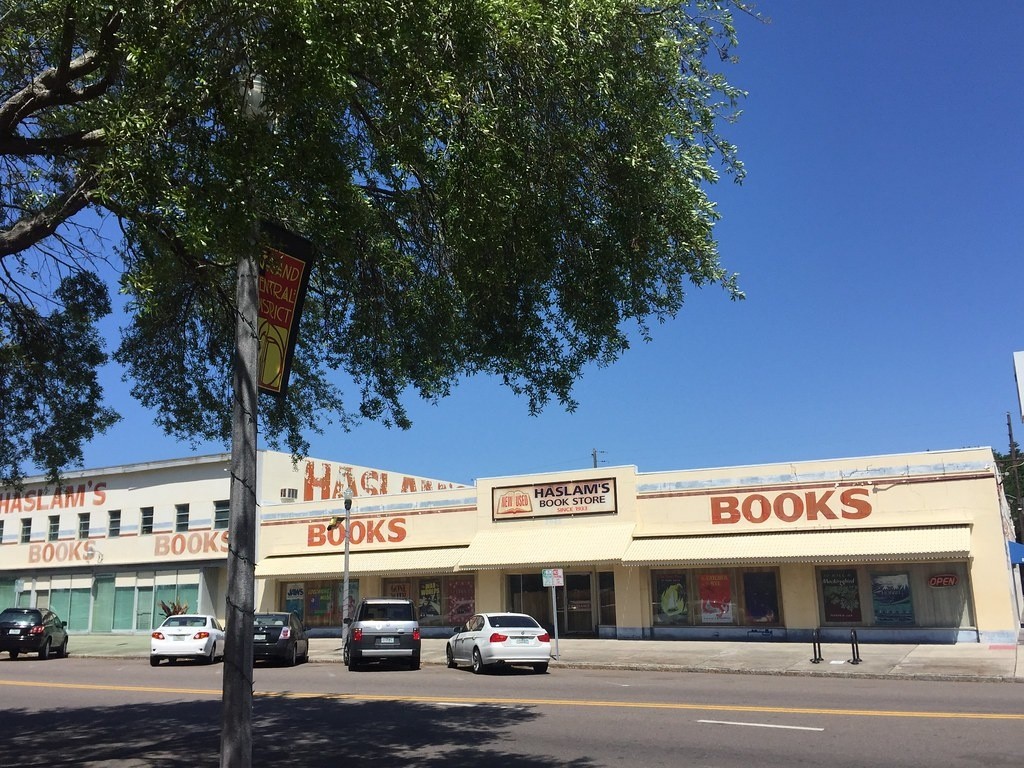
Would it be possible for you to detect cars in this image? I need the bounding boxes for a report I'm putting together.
[445,612,551,674]
[0,608,68,659]
[253,612,309,666]
[150,614,226,665]
[653,600,773,623]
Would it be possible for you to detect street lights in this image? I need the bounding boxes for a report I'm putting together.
[342,485,353,646]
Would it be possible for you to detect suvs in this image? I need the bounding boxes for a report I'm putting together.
[343,598,421,671]
